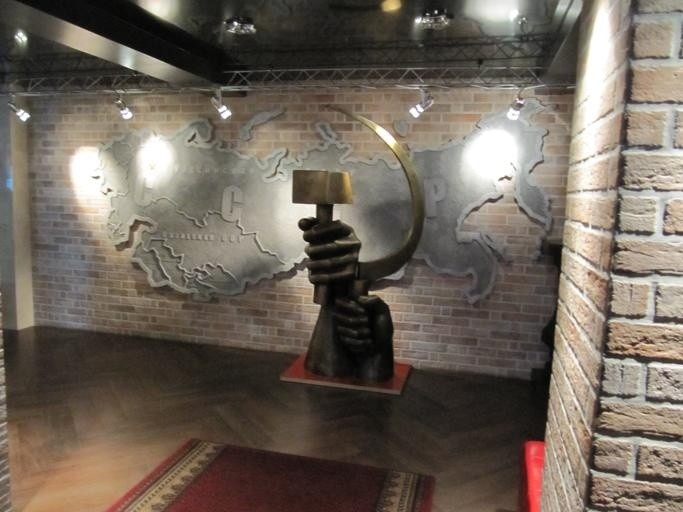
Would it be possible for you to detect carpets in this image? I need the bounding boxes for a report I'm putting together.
[105,437,435,511]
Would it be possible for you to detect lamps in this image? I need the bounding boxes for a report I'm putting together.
[506,88,526,121]
[408,86,434,118]
[112,93,132,121]
[209,91,231,119]
[4,95,30,123]
